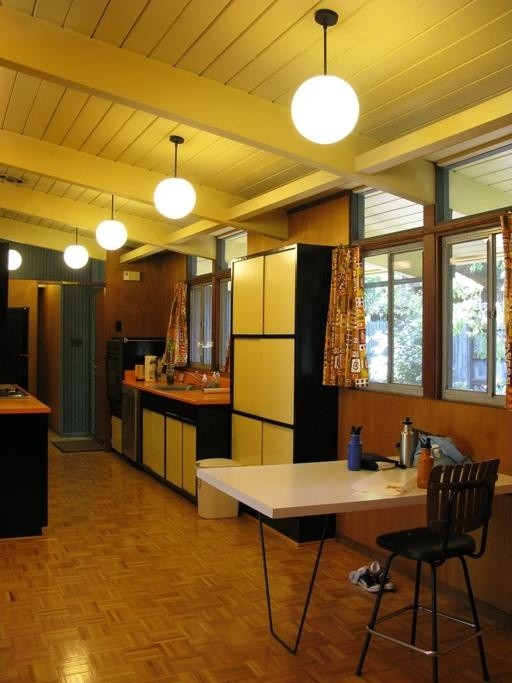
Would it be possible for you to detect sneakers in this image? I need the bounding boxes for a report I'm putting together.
[357,559,395,593]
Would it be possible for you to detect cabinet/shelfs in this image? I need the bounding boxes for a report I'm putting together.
[142,408,164,481]
[229,243,338,547]
[111,415,122,453]
[165,416,197,503]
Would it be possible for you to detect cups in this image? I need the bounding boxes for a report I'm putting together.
[166,365,176,385]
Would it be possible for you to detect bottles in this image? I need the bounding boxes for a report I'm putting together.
[416,438,433,489]
[202,374,208,391]
[348,424,364,471]
[399,416,415,468]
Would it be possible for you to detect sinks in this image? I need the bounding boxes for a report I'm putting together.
[151,385,200,391]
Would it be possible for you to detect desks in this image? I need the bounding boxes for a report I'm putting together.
[195,458,512,653]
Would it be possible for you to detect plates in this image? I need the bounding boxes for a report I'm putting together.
[8,390,23,398]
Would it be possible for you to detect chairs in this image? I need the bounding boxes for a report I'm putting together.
[355,460,499,683]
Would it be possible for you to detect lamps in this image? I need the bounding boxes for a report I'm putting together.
[290,9,360,144]
[154,136,196,219]
[8,196,127,271]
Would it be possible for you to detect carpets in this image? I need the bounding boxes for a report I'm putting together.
[50,439,106,453]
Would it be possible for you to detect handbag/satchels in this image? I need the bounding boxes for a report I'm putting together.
[412,432,475,469]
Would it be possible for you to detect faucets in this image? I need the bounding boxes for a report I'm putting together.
[184,371,204,387]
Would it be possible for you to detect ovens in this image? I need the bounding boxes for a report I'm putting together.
[104,336,174,418]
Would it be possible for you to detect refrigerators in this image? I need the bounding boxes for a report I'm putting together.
[120,385,145,464]
